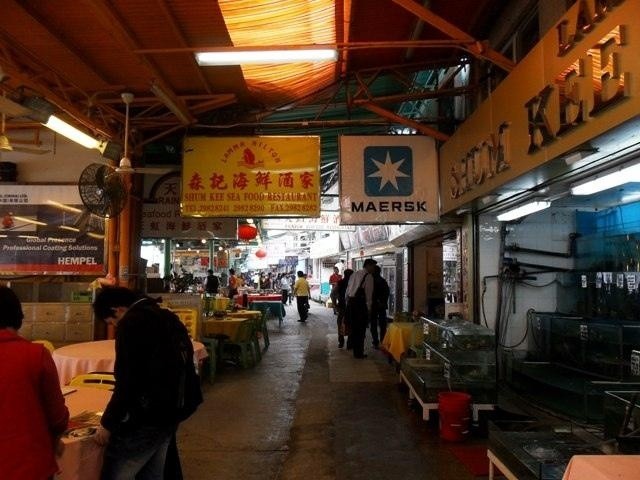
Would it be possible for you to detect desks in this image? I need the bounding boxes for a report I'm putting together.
[227,309,261,322]
[239,291,282,303]
[251,301,285,331]
[382,322,422,369]
[205,318,249,341]
[52,340,208,386]
[53,386,113,480]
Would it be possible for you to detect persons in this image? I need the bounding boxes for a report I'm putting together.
[344,257,377,359]
[240,270,295,307]
[292,270,312,323]
[329,265,342,316]
[336,269,353,349]
[369,265,390,348]
[1,285,70,476]
[94,283,203,476]
[305,275,312,316]
[227,267,238,300]
[203,269,219,317]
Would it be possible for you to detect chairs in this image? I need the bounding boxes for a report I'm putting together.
[219,318,257,370]
[255,306,271,348]
[199,337,216,384]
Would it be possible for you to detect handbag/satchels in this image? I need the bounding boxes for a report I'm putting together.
[354,273,368,302]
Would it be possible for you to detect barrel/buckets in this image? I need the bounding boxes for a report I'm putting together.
[438,392,471,443]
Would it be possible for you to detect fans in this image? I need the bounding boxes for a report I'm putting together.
[70,162,128,239]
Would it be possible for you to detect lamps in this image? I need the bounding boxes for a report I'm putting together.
[115,91,135,172]
